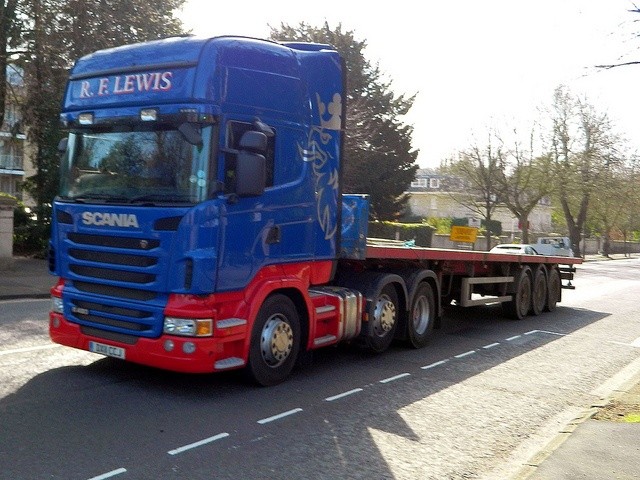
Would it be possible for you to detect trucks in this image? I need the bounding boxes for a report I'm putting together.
[50,36,582,388]
[537,237,574,258]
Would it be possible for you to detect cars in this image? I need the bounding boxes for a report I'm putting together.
[489,244,539,256]
